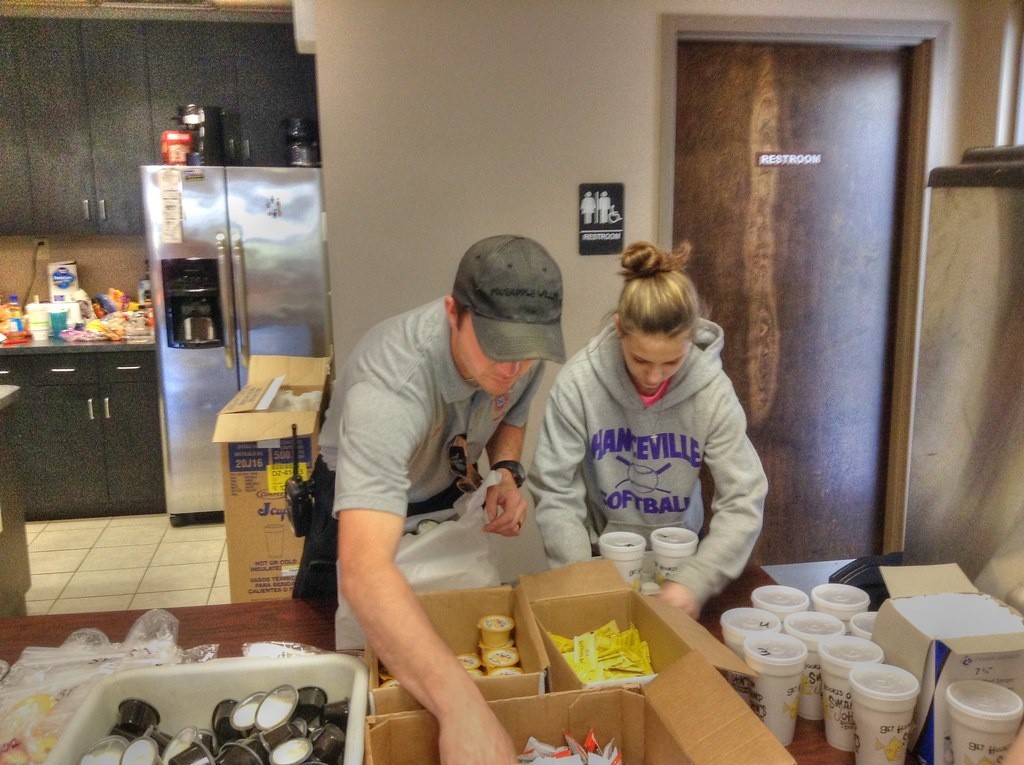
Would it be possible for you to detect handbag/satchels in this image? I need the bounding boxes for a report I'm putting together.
[335,470,503,650]
[829,557,891,612]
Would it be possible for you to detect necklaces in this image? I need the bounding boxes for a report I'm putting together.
[639,392,663,446]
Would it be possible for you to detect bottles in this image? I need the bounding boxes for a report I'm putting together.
[139,260,152,306]
[7,295,24,340]
[290,119,309,166]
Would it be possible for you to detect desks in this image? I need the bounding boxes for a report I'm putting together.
[0,563,857,765]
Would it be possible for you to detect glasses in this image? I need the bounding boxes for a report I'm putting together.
[447,437,482,493]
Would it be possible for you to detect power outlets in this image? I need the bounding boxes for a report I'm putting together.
[34,239,49,260]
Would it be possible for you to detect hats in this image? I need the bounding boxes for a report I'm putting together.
[452,234,568,365]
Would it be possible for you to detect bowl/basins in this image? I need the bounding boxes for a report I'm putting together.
[76,685,350,765]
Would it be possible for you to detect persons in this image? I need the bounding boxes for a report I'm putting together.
[292,233,566,765]
[525,240,768,621]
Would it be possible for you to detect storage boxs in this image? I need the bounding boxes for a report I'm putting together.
[519,559,758,696]
[213,345,335,604]
[41,653,368,765]
[869,562,1024,765]
[366,585,553,716]
[363,649,799,764]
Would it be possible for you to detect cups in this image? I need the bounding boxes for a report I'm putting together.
[51,311,67,339]
[649,527,699,584]
[811,584,870,636]
[186,153,200,165]
[599,532,647,592]
[819,636,884,752]
[851,612,878,640]
[743,633,807,746]
[946,680,1024,765]
[784,612,845,720]
[26,304,48,340]
[721,607,781,710]
[848,663,921,765]
[751,585,810,634]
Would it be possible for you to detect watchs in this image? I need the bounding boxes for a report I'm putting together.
[491,460,526,488]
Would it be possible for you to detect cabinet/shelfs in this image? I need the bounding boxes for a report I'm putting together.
[0,17,322,236]
[0,350,166,619]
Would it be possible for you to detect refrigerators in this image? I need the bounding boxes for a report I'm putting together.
[140,164,330,525]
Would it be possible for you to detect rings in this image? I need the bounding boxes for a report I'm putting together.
[518,521,522,529]
